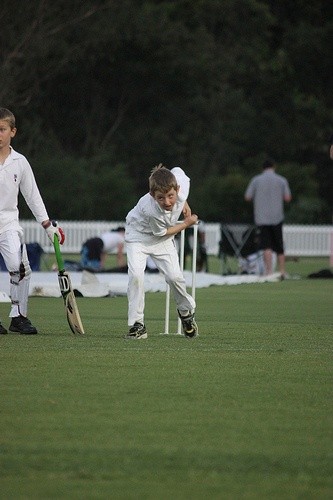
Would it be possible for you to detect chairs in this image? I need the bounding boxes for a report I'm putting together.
[234,228,264,275]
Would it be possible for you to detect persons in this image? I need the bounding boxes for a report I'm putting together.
[123,163,198,340]
[52,227,125,273]
[0,108,65,334]
[244,160,292,278]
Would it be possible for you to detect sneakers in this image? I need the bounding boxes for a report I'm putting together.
[9,315,37,334]
[127,322,147,339]
[0,322,7,333]
[177,309,198,339]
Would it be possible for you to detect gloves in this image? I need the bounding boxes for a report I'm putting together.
[43,221,65,245]
